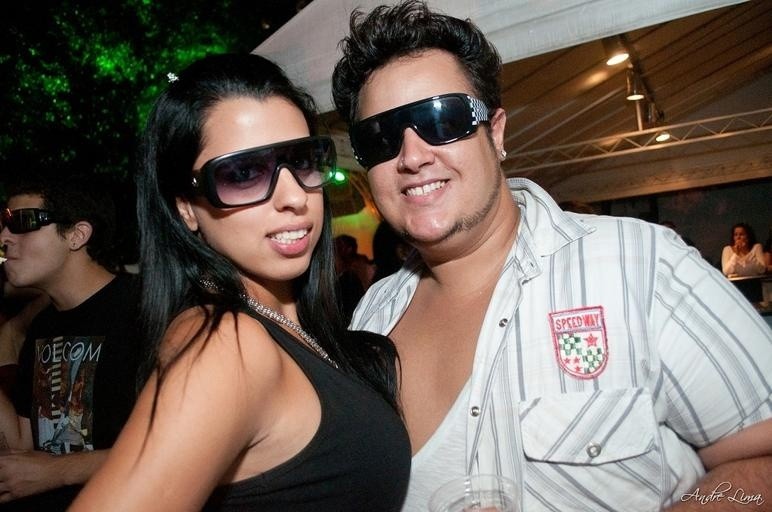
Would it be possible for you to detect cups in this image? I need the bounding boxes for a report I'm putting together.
[428,473,522,512]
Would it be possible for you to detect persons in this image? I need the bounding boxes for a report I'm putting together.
[330,253,367,327]
[721,221,765,279]
[367,219,409,289]
[74,46,413,511]
[0,179,146,511]
[331,234,376,290]
[334,0,771,512]
[1,260,55,370]
[761,228,771,308]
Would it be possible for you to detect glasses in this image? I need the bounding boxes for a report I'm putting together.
[0,206,61,235]
[346,92,491,169]
[161,135,338,209]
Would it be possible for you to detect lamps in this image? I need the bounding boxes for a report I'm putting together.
[602,34,658,125]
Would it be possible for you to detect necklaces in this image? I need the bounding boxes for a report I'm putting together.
[197,279,340,371]
